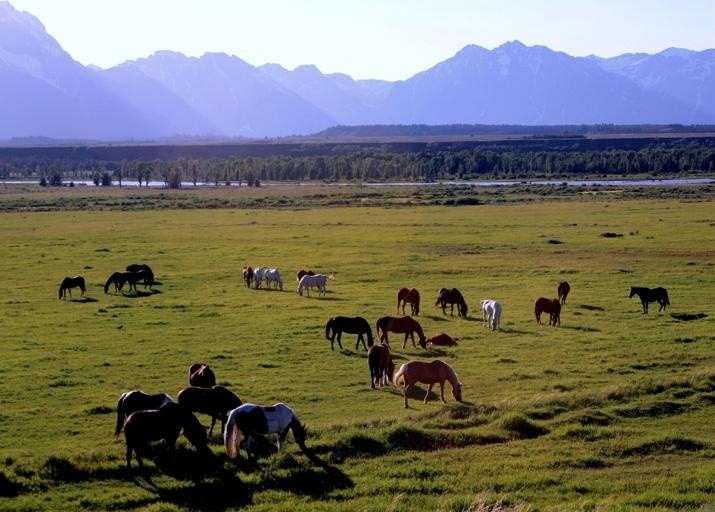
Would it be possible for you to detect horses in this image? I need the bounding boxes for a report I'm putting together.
[243,265,284,293]
[297,270,323,293]
[629,284,673,317]
[325,315,376,352]
[435,287,468,319]
[394,360,464,409]
[104,263,156,296]
[554,278,574,298]
[376,313,426,349]
[532,296,559,328]
[297,273,336,298]
[112,362,312,477]
[367,340,397,389]
[55,275,88,298]
[480,298,503,331]
[396,286,421,317]
